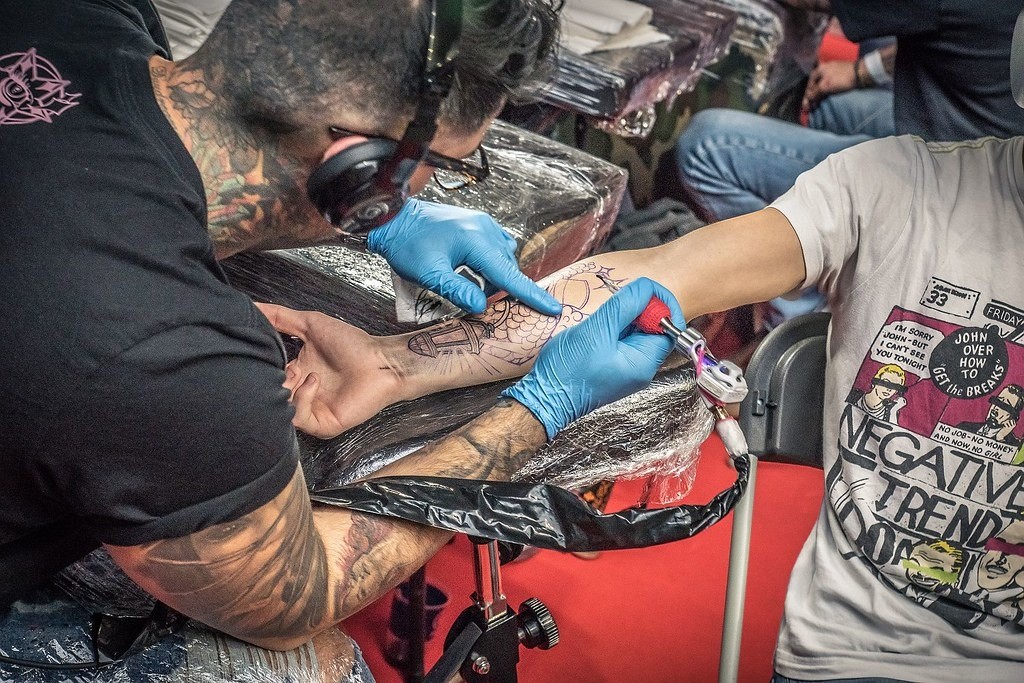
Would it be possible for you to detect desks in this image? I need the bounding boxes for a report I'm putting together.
[522,1,737,137]
[211,116,630,340]
[295,343,713,502]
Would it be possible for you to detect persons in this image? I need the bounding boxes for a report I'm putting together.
[0,0,682,652]
[246,13,1024,683]
[674,0,1024,337]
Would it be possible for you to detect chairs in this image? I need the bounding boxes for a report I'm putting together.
[717,312,832,683]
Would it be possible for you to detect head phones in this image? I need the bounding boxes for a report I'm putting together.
[305,0,464,235]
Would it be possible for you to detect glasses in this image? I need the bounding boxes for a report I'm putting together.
[327,126,491,192]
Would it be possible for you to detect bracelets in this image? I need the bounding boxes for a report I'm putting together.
[854,60,863,89]
[863,49,892,85]
